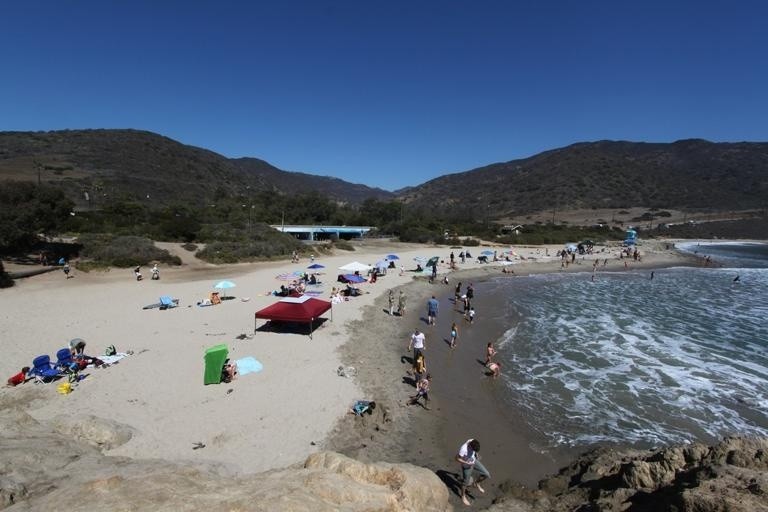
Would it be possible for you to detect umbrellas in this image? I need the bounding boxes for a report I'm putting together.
[213,281,236,299]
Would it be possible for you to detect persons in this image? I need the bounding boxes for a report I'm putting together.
[39,250,72,279]
[734,275,741,282]
[150,263,160,279]
[134,266,143,281]
[71,343,93,365]
[1,367,33,388]
[209,291,222,305]
[222,360,237,383]
[455,438,491,505]
[276,242,715,417]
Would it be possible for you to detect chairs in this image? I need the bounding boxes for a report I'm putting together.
[160,296,177,308]
[27,348,76,385]
